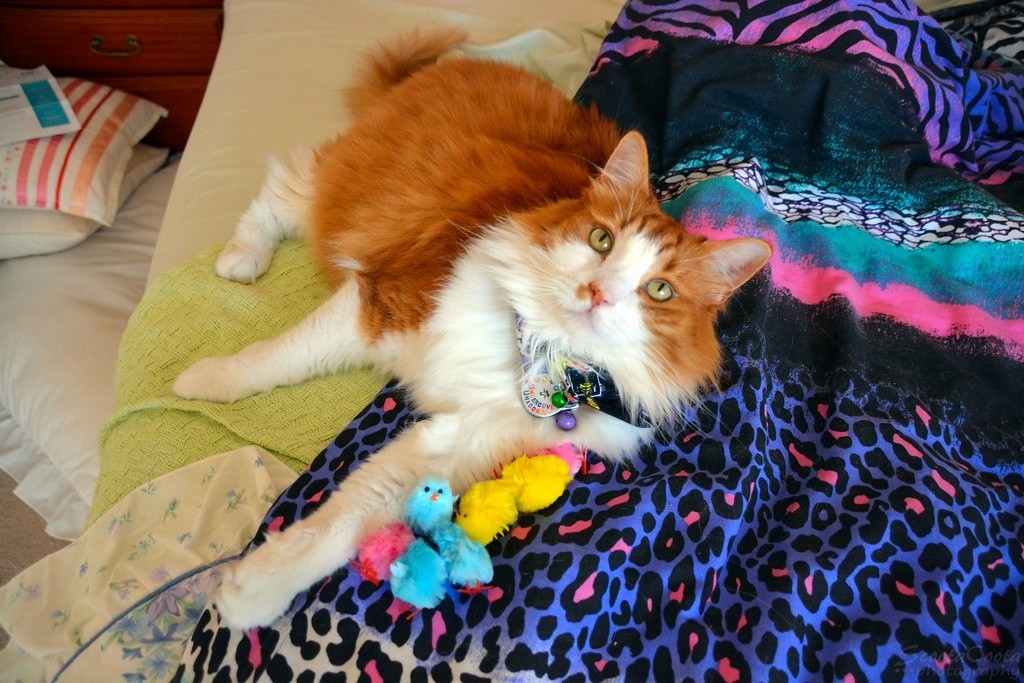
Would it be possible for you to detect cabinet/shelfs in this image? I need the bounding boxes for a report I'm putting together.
[0,0,225,155]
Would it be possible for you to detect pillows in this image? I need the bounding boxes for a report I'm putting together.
[1,62,172,229]
[0,156,183,545]
[1,144,171,261]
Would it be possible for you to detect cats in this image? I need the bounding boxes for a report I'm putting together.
[172,23,773,633]
